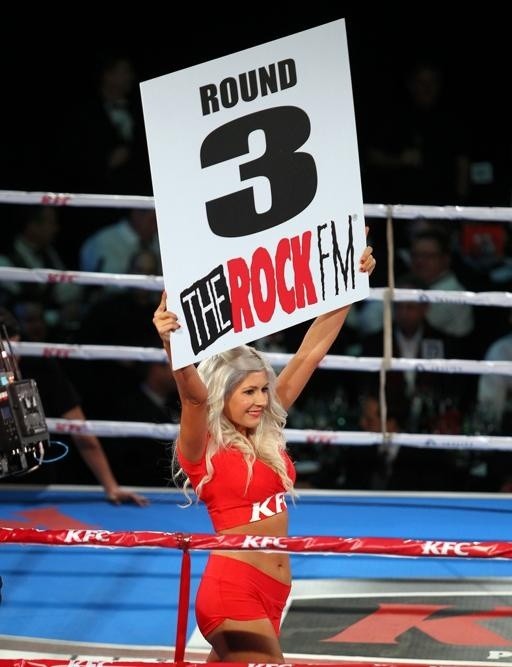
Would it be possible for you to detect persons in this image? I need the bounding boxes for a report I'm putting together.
[0,68,512,508]
[153,227,377,664]
